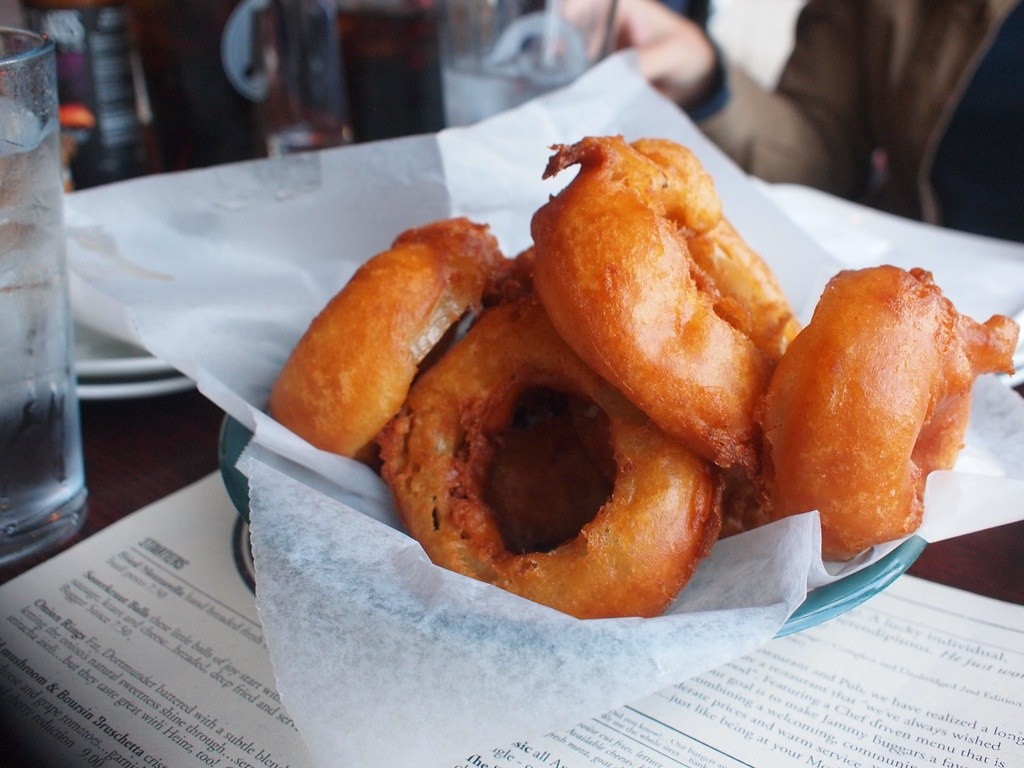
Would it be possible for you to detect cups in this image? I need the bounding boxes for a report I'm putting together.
[437,0,618,129]
[257,0,351,155]
[0,26,89,564]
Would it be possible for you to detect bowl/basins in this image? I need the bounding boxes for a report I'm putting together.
[216,412,924,642]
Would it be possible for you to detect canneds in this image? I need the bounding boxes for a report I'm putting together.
[30,0,143,156]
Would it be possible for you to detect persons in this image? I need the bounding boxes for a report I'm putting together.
[560,0,1024,245]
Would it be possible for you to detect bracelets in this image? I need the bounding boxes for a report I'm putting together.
[683,37,731,123]
